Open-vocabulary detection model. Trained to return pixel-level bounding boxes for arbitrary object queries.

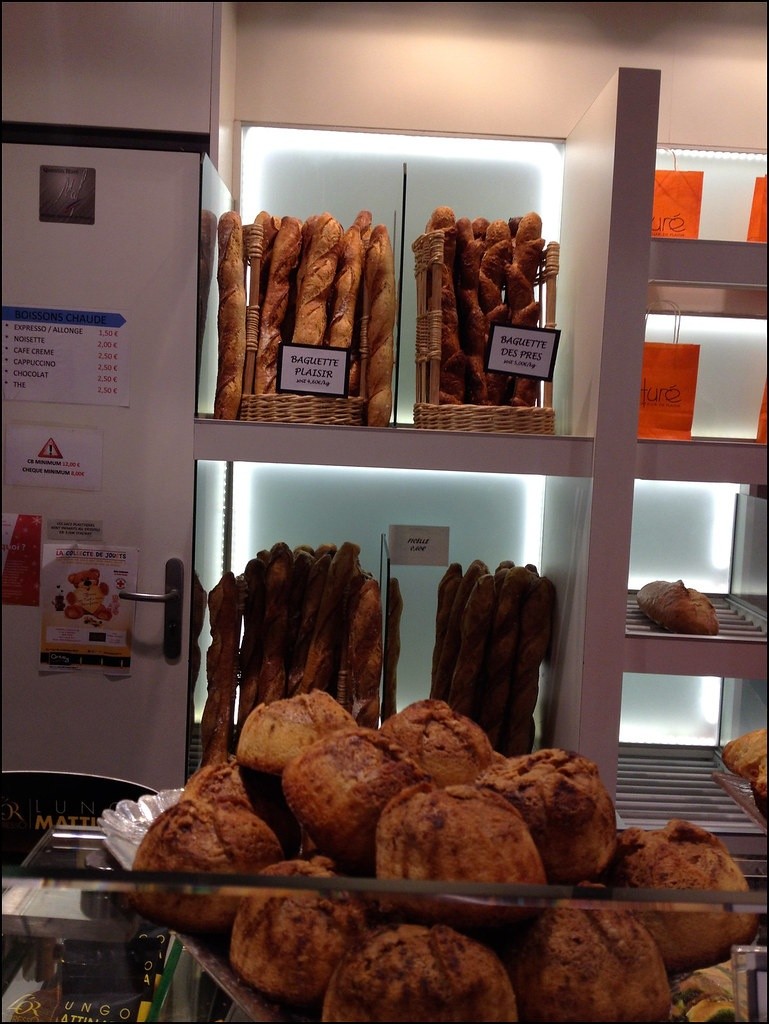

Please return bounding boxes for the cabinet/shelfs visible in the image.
[195,65,769,860]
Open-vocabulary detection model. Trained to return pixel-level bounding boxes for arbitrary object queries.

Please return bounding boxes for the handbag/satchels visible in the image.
[637,299,702,441]
[651,146,705,239]
[746,175,767,244]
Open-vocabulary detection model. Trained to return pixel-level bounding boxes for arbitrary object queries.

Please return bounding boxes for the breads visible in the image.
[637,579,718,637]
[424,205,545,409]
[127,690,751,1021]
[212,208,395,428]
[196,541,403,769]
[426,557,559,757]
[721,727,766,805]
